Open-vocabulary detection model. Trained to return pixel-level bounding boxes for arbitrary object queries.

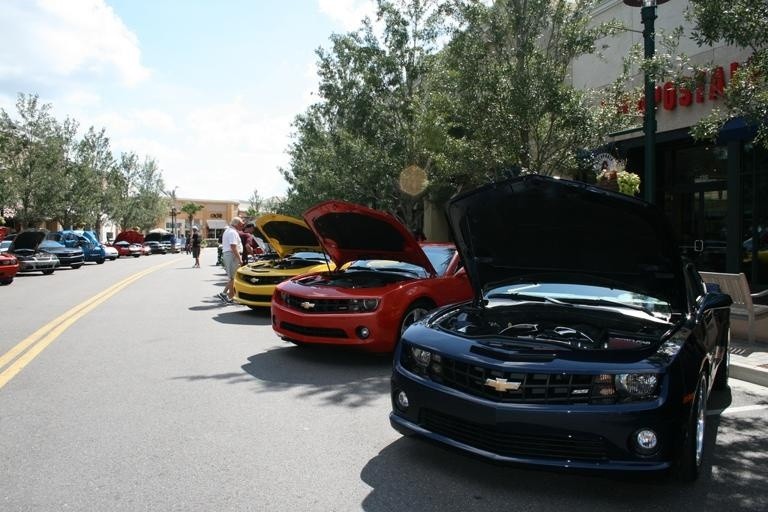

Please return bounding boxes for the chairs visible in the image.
[696,271,767,347]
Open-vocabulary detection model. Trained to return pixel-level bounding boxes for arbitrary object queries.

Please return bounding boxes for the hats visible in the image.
[232,216,245,227]
[192,225,198,231]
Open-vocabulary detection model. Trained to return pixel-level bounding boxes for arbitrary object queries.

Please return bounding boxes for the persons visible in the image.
[238,221,258,266]
[180,233,193,255]
[216,216,245,306]
[189,227,201,269]
[170,234,176,253]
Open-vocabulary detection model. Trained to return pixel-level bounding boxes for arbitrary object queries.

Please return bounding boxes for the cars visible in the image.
[232,213,337,312]
[0,228,184,285]
[269,199,476,362]
[389,173,733,484]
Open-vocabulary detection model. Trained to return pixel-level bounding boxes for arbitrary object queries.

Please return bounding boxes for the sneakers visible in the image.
[218,293,236,304]
